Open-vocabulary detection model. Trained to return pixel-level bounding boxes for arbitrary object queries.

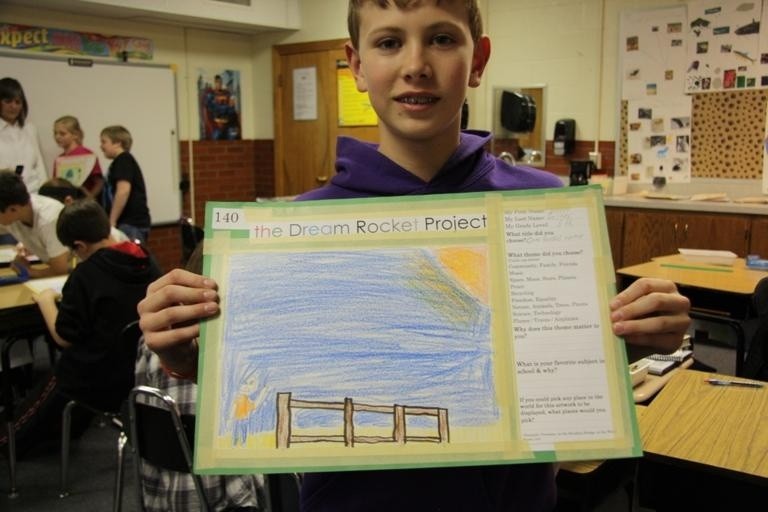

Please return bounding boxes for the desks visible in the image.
[557,360,767,511]
[615,248,768,377]
[0,274,67,342]
[0,236,75,284]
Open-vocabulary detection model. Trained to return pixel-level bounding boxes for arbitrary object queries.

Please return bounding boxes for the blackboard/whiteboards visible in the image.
[0,47,183,229]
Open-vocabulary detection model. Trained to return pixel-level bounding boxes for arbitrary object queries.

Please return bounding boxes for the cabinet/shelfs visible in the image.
[749,216,768,262]
[604,205,624,293]
[622,206,751,269]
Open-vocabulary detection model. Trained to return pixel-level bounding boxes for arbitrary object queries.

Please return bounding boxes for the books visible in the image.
[646,336,691,376]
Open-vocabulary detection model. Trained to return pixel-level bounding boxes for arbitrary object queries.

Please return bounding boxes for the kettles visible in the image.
[569,159,592,187]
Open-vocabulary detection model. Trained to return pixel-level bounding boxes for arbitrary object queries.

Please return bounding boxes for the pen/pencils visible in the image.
[72,256,77,269]
[703,378,763,388]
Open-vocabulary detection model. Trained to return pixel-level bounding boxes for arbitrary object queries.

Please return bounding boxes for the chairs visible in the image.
[115,386,213,511]
[60,319,139,501]
[1,309,52,501]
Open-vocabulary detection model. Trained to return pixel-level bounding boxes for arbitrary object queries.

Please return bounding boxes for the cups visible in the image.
[701,77,711,89]
[723,69,768,89]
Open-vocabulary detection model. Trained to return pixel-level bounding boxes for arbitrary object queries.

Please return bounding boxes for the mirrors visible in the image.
[485,79,549,169]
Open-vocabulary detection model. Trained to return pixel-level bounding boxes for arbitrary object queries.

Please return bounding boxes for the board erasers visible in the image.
[67,58,95,67]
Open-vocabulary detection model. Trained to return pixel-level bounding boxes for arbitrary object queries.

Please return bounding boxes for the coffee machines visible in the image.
[553,118,575,156]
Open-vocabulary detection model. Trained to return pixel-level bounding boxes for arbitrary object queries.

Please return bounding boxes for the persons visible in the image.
[0,77,48,194]
[1,168,132,280]
[52,116,105,200]
[101,125,151,240]
[201,75,239,142]
[38,176,93,205]
[0,202,162,457]
[136,236,272,512]
[136,0,693,512]
